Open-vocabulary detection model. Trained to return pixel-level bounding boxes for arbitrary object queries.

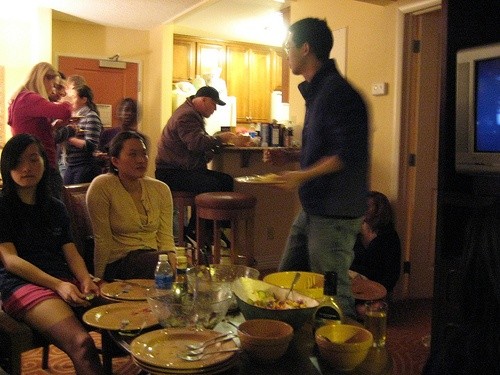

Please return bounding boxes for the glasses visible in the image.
[285,47,295,54]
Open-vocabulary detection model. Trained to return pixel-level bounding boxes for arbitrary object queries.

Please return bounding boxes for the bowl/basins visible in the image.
[186,264,260,297]
[353,280,387,305]
[314,324,373,371]
[237,319,293,364]
[230,136,250,147]
[231,277,319,330]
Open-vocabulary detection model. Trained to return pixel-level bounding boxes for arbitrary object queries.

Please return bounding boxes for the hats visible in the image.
[196,86,226,106]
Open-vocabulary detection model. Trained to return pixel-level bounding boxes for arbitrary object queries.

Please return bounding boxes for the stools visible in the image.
[171,190,198,247]
[195,192,258,267]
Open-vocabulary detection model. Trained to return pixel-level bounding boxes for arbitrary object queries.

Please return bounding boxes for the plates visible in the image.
[129,328,240,375]
[262,271,326,301]
[83,302,159,330]
[233,176,287,184]
[100,279,156,302]
[71,117,85,119]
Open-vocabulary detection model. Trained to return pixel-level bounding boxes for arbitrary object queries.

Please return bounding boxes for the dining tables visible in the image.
[102,310,393,375]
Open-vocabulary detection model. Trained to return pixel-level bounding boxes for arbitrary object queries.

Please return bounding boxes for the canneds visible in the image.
[259,123,283,147]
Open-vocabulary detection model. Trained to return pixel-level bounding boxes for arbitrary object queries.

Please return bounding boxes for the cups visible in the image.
[364,301,387,347]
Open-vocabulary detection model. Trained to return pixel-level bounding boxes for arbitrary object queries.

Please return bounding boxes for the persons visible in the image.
[85,127,177,281]
[348,191,402,311]
[94,98,146,174]
[263,18,370,321]
[155,86,233,248]
[0,133,110,375]
[9,62,102,186]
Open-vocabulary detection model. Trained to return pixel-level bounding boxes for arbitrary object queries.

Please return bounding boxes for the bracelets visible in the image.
[53,280,63,290]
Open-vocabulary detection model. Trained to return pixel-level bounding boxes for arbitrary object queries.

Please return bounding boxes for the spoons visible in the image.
[187,330,232,349]
[187,335,235,355]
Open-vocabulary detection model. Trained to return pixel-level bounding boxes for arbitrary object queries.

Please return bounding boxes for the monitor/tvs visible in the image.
[452,42,500,175]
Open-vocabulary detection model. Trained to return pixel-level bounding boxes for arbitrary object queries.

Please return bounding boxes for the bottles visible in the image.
[261,122,293,147]
[154,254,173,290]
[175,247,188,284]
[312,272,344,341]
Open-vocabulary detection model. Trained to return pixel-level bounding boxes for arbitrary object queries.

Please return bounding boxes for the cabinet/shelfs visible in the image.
[271,46,283,92]
[431,167,500,375]
[173,34,227,84]
[226,40,271,124]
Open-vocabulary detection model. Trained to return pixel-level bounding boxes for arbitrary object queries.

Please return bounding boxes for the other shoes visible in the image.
[186,231,197,248]
[220,232,230,248]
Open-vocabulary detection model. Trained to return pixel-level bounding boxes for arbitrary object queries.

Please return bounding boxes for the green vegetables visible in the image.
[246,290,278,309]
[164,294,222,330]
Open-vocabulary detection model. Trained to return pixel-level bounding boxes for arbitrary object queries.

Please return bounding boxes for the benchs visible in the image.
[0,182,97,375]
